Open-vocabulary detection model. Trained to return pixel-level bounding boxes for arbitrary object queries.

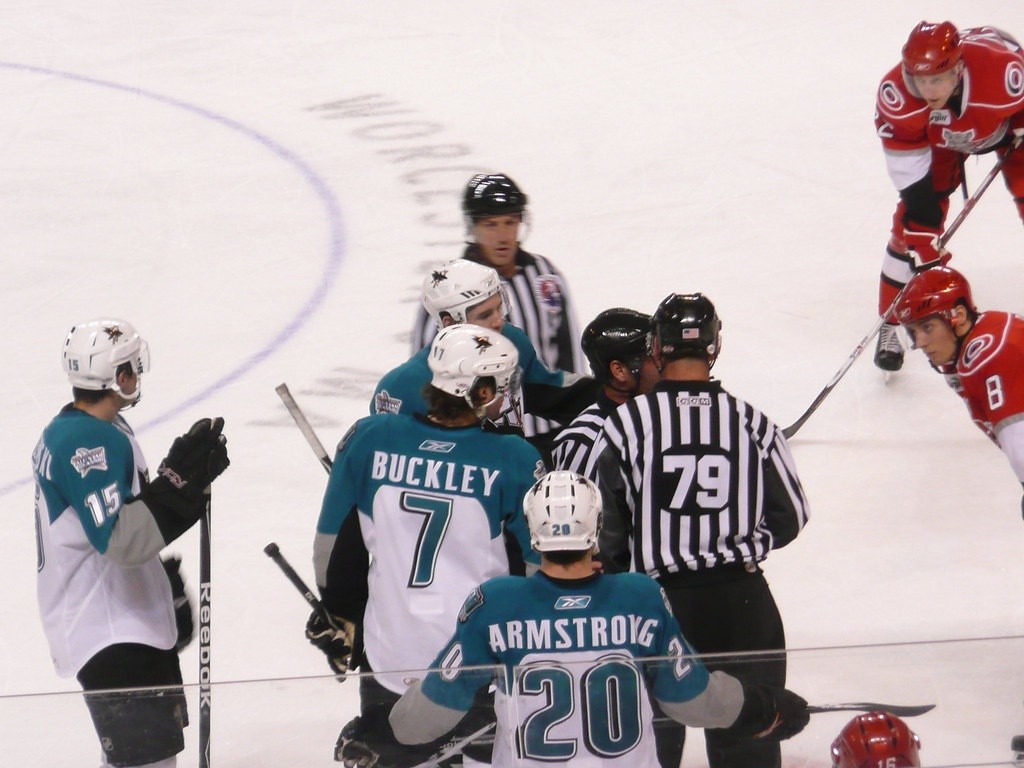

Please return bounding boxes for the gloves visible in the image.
[901,226,951,272]
[157,416,230,500]
[305,601,353,682]
[725,684,809,743]
[163,558,194,655]
[334,705,411,768]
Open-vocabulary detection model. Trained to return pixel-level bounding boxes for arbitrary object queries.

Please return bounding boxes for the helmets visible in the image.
[421,258,500,328]
[63,321,143,402]
[463,173,526,218]
[830,711,919,768]
[427,323,519,397]
[892,265,974,330]
[580,308,655,374]
[523,472,603,555]
[649,291,723,362]
[902,21,962,77]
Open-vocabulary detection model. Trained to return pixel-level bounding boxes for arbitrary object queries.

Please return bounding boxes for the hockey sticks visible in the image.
[448,704,937,749]
[276,383,333,476]
[781,137,1023,441]
[198,484,212,768]
[265,540,338,629]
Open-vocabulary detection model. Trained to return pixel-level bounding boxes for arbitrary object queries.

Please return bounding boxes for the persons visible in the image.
[334,470,811,768]
[33,317,230,768]
[549,309,657,473]
[367,259,583,420]
[305,324,547,768]
[411,174,590,451]
[894,266,1024,520]
[831,710,921,768]
[873,21,1024,386]
[585,293,810,768]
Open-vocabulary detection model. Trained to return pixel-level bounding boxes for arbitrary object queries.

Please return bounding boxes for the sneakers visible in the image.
[874,324,903,382]
